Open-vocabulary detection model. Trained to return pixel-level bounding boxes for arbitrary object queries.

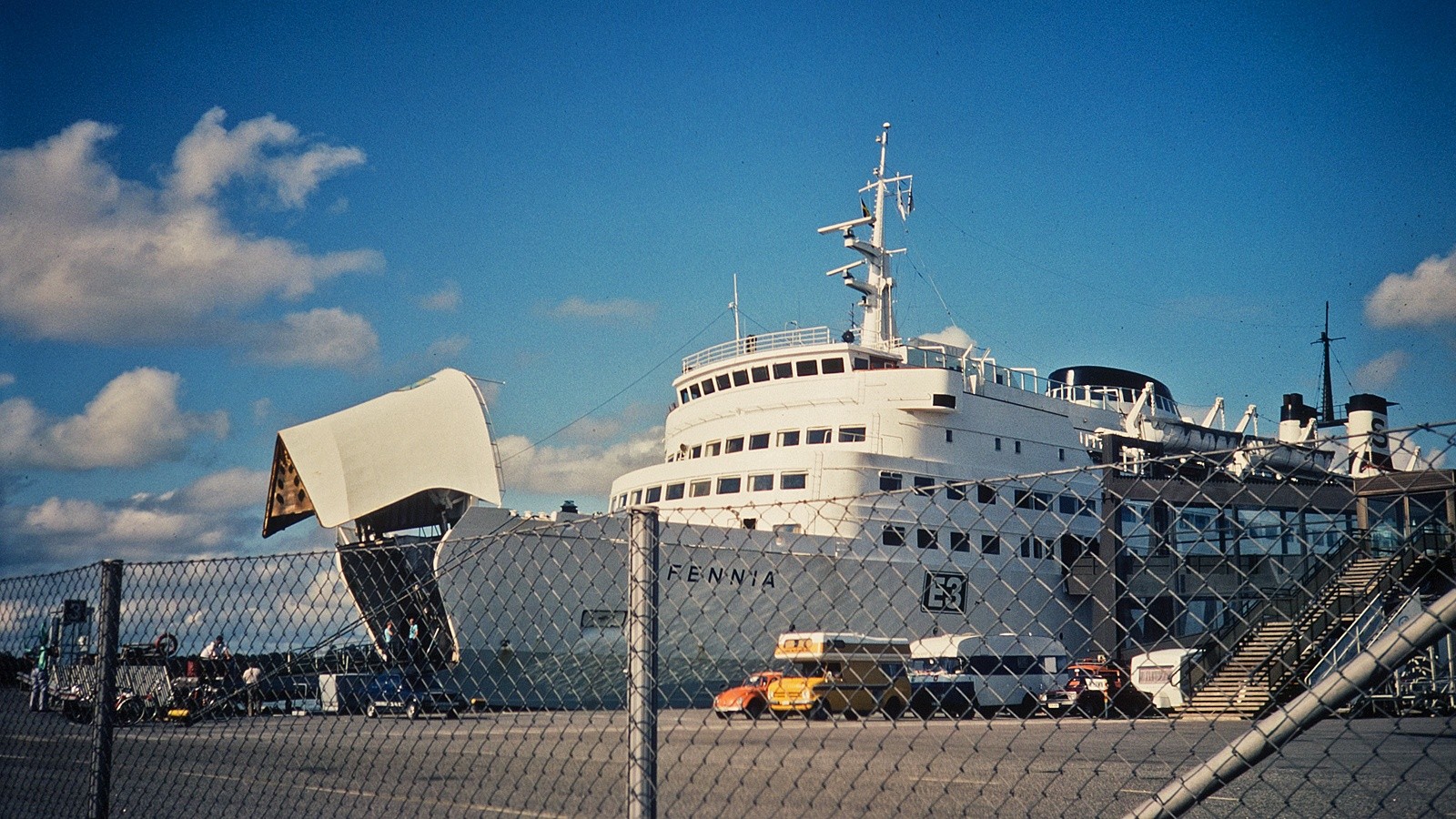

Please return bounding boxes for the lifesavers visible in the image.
[155,633,178,656]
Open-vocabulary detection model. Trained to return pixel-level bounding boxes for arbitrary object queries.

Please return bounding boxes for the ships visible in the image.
[259,122,1456,712]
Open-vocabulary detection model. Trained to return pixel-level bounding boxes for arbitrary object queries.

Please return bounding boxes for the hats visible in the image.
[40,634,48,643]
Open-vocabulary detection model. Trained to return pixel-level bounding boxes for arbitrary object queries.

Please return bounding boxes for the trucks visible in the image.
[764,632,913,721]
[1038,654,1146,720]
[908,632,1071,721]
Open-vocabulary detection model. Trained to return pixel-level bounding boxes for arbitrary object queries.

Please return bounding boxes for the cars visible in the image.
[357,662,470,721]
[713,671,783,719]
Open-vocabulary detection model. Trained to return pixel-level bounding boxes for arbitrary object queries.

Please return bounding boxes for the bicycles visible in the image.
[51,668,238,727]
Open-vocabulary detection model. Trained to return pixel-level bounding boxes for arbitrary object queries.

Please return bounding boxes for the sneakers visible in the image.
[40,710,52,714]
[30,709,40,714]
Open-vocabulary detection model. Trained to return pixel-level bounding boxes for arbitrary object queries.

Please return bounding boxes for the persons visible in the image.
[24,634,59,712]
[384,620,393,661]
[242,662,264,717]
[408,617,418,660]
[200,636,230,660]
